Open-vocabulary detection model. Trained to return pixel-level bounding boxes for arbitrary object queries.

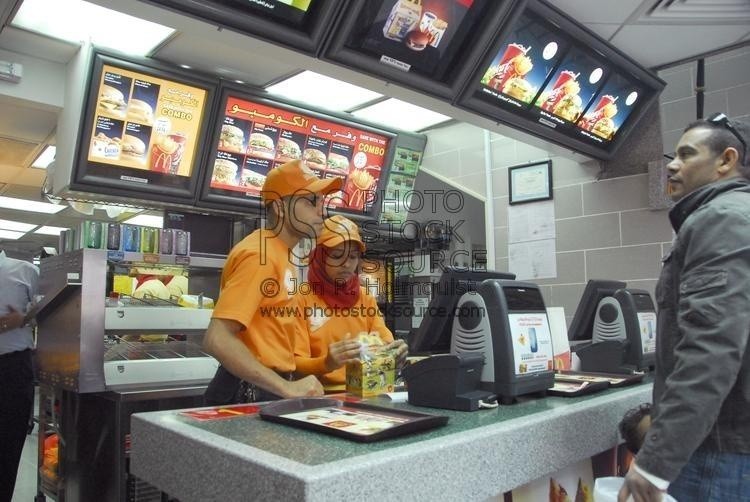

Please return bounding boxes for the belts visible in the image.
[278,373,295,382]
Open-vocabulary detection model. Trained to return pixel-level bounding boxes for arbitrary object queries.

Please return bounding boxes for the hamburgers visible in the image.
[404,31,429,51]
[593,119,615,139]
[552,90,582,120]
[504,78,532,102]
[212,124,351,206]
[87,84,152,167]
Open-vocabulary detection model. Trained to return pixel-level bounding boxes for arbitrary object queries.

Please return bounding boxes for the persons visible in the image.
[1,245,41,501]
[615,111,750,502]
[201,160,325,407]
[294,215,410,396]
[618,402,658,454]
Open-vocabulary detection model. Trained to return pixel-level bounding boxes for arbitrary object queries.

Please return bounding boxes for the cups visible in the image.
[528,327,539,353]
[583,93,615,132]
[540,69,577,115]
[648,320,653,339]
[488,43,527,95]
[420,11,438,32]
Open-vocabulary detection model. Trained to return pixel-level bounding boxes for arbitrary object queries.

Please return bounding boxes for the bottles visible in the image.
[58,219,191,256]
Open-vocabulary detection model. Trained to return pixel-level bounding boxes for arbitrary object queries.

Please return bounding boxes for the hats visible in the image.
[261,161,344,206]
[314,215,366,255]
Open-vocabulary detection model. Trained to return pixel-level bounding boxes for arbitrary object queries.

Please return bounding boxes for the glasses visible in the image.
[708,110,747,166]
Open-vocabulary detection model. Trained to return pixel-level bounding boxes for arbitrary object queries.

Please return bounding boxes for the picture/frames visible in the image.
[507,160,553,205]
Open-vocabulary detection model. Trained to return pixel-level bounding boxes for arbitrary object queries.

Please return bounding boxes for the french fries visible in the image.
[603,101,618,119]
[433,18,447,30]
[348,168,373,188]
[152,129,179,154]
[564,80,581,95]
[514,56,534,75]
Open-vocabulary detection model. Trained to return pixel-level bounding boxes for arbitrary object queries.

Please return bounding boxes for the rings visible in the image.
[3,324,8,330]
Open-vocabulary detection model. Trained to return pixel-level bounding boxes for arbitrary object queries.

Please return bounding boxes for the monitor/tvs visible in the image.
[567,280,627,341]
[406,266,516,357]
